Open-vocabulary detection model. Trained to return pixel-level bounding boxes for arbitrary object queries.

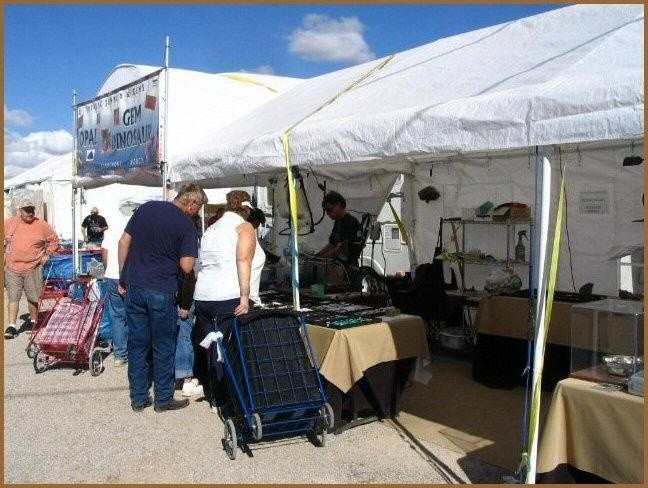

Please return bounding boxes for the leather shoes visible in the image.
[153,398,190,413]
[131,396,152,412]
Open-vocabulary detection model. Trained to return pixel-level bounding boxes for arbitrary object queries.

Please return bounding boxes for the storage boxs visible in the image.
[437,326,473,349]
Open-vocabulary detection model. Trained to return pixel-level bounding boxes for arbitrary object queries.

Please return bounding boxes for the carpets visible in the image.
[353,351,553,475]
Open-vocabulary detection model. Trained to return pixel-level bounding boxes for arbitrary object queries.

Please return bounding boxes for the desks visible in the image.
[472,289,645,385]
[252,289,431,434]
[534,354,644,483]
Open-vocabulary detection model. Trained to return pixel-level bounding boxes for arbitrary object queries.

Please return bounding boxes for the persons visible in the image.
[114,181,208,413]
[243,206,267,232]
[188,190,268,415]
[102,203,134,366]
[174,211,204,399]
[315,189,367,270]
[80,206,109,248]
[3,196,60,341]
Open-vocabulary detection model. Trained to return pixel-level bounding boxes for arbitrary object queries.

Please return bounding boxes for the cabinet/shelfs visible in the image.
[436,217,531,316]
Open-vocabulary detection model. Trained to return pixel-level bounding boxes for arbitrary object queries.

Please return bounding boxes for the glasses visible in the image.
[326,204,338,213]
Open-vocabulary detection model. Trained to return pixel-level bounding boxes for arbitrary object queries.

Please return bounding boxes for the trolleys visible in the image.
[30,261,116,377]
[213,306,336,459]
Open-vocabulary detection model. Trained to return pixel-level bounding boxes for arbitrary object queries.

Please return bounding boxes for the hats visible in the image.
[242,201,253,209]
[19,198,35,209]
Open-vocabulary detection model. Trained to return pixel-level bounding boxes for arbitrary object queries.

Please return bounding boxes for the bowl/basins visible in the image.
[600,354,643,376]
[439,327,468,349]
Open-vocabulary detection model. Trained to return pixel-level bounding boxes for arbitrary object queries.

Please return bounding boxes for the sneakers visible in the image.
[4,326,19,338]
[25,321,39,334]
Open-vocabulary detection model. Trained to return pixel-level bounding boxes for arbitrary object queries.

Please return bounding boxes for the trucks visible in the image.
[359,174,410,295]
